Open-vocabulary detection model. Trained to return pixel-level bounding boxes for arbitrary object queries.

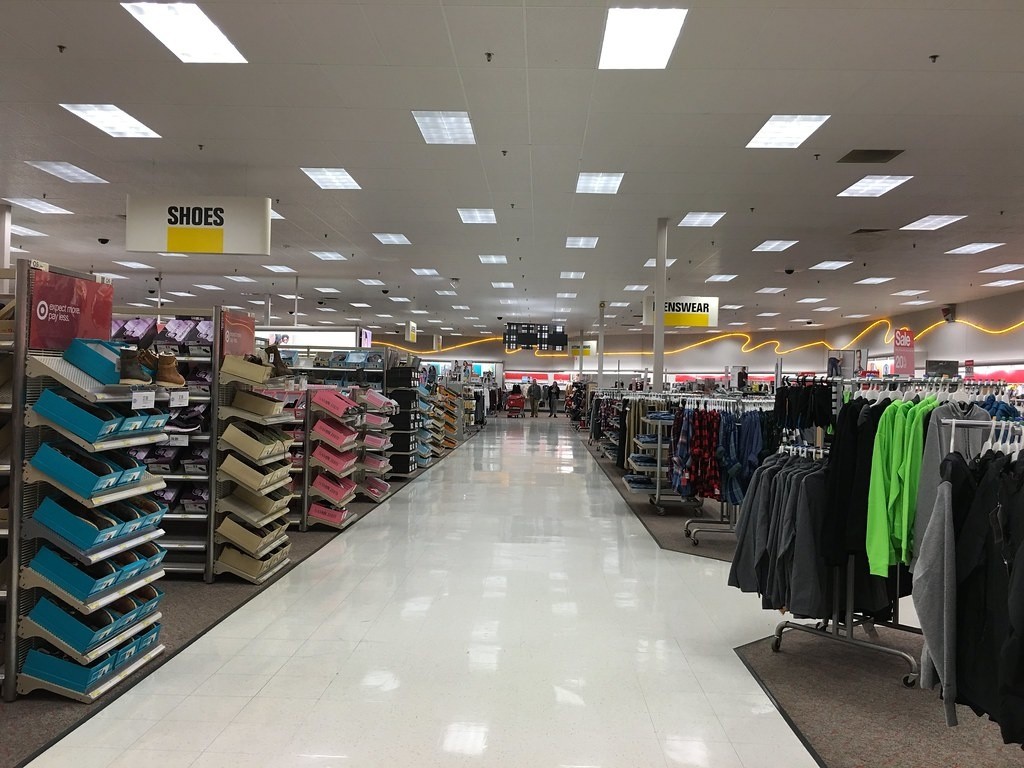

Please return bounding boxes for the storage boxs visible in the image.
[20,316,459,696]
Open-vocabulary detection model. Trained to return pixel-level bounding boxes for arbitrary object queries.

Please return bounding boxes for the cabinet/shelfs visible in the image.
[434,393,446,457]
[622,410,705,516]
[417,389,434,469]
[0,259,188,705]
[339,384,400,504]
[254,364,359,532]
[439,385,459,449]
[112,304,291,585]
[277,345,427,480]
[597,402,624,459]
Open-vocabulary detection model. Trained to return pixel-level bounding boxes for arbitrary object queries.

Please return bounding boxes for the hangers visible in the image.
[948,418,957,453]
[778,442,825,461]
[783,374,1024,416]
[980,417,1024,462]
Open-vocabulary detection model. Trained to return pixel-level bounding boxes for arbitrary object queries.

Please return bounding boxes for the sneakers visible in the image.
[159,406,200,432]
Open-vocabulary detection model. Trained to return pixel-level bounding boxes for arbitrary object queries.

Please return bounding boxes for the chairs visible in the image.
[590,385,775,417]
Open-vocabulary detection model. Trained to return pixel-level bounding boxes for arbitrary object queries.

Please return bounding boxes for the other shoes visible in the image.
[535,414,538,417]
[554,415,558,418]
[27,388,170,668]
[549,414,553,417]
[530,413,534,417]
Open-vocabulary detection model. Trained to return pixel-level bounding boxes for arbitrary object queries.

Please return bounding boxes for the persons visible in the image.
[829,351,843,376]
[854,350,864,372]
[454,360,460,371]
[527,379,541,418]
[428,366,436,383]
[547,381,560,418]
[738,366,748,390]
[463,361,470,378]
[420,368,427,383]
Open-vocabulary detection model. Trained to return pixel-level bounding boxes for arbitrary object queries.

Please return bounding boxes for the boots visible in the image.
[137,348,159,372]
[155,350,185,388]
[119,346,153,386]
